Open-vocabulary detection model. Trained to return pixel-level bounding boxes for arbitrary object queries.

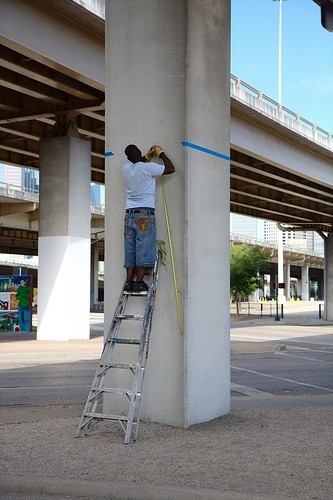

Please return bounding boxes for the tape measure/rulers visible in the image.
[151,146,183,334]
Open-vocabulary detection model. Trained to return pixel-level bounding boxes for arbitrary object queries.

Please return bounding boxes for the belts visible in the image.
[125,209,154,215]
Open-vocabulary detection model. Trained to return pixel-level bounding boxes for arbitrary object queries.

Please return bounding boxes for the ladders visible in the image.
[76,240,165,445]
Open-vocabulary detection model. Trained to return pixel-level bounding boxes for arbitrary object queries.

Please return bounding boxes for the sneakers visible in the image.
[122,280,135,294]
[132,280,149,296]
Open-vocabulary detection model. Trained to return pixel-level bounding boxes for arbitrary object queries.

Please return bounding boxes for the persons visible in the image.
[122,144,175,293]
[15,279,30,332]
